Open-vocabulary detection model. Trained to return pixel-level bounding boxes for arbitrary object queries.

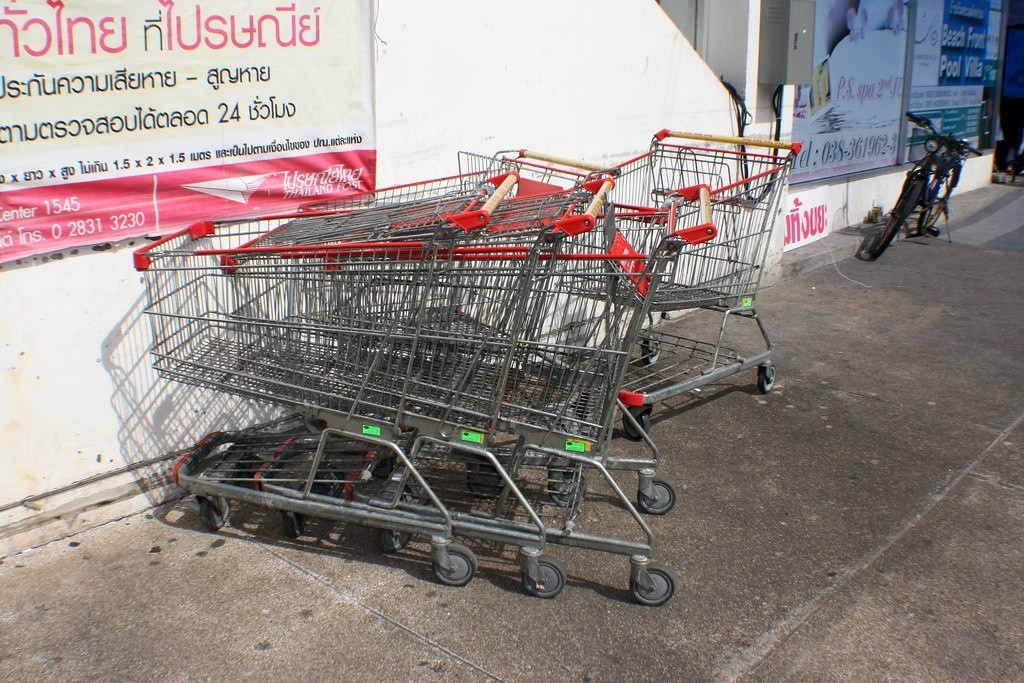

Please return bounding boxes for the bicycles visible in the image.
[865,111,986,255]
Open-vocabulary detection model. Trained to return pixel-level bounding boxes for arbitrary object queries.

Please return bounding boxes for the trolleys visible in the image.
[133,130,804,604]
